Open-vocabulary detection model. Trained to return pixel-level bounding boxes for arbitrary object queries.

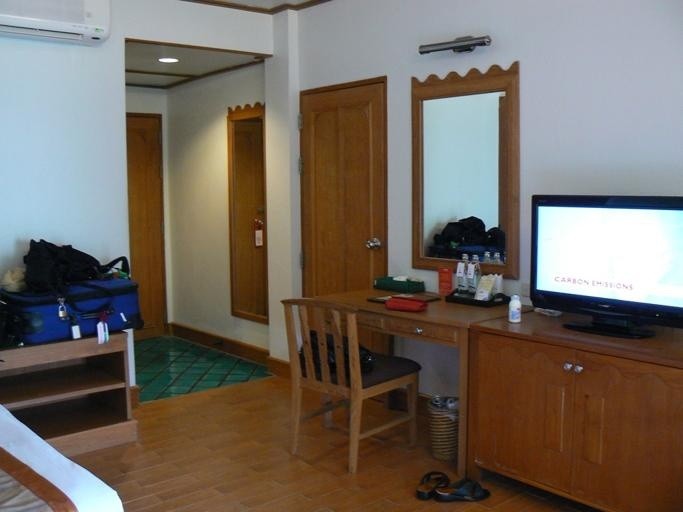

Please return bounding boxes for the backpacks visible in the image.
[20,239,130,316]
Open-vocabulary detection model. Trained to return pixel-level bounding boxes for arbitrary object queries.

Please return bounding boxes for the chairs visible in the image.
[279,298,422,475]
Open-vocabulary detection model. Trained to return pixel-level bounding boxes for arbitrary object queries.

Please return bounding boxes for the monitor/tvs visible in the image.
[530,194,683,340]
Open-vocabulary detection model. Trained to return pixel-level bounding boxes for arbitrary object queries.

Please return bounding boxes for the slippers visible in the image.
[431,477,491,503]
[415,471,450,501]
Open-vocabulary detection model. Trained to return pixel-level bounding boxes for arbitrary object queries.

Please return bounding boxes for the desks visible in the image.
[314,288,533,479]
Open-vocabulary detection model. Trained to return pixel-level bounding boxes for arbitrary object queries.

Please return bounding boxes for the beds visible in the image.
[0,403,125,512]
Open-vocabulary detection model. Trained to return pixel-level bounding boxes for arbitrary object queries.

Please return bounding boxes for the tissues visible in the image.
[375,275,425,293]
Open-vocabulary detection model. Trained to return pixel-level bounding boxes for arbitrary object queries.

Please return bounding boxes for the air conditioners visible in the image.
[1,0,111,47]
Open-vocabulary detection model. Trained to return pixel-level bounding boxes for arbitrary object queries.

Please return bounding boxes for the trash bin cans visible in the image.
[426,396,460,461]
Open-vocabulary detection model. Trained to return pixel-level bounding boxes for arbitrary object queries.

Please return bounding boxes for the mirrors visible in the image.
[409,60,521,280]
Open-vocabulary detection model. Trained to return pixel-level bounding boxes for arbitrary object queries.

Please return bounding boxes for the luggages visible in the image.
[428,245,505,263]
[0,267,143,347]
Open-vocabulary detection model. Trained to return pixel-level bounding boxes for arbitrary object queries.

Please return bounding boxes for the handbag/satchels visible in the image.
[434,216,505,246]
[301,329,376,372]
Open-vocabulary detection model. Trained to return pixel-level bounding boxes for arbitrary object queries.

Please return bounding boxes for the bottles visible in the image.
[455,253,481,293]
[507,294,522,324]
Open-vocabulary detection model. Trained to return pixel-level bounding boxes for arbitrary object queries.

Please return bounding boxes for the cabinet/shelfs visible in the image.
[0,332,138,458]
[467,308,683,512]
